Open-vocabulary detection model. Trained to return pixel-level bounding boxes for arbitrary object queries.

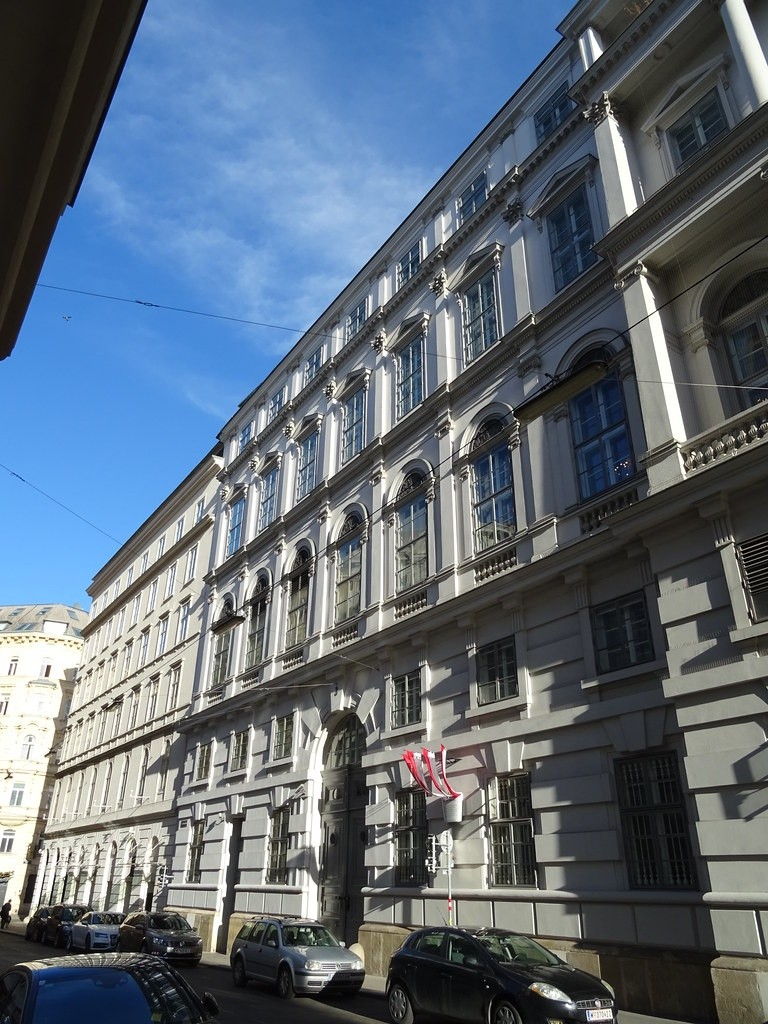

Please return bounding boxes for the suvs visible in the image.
[45,904,103,950]
[119,910,204,971]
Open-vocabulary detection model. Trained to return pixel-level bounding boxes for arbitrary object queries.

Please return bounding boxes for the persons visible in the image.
[0,899,12,929]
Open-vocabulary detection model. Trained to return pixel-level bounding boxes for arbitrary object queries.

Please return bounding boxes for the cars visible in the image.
[229,915,367,1002]
[66,911,126,955]
[0,950,222,1024]
[26,906,73,945]
[386,923,620,1024]
